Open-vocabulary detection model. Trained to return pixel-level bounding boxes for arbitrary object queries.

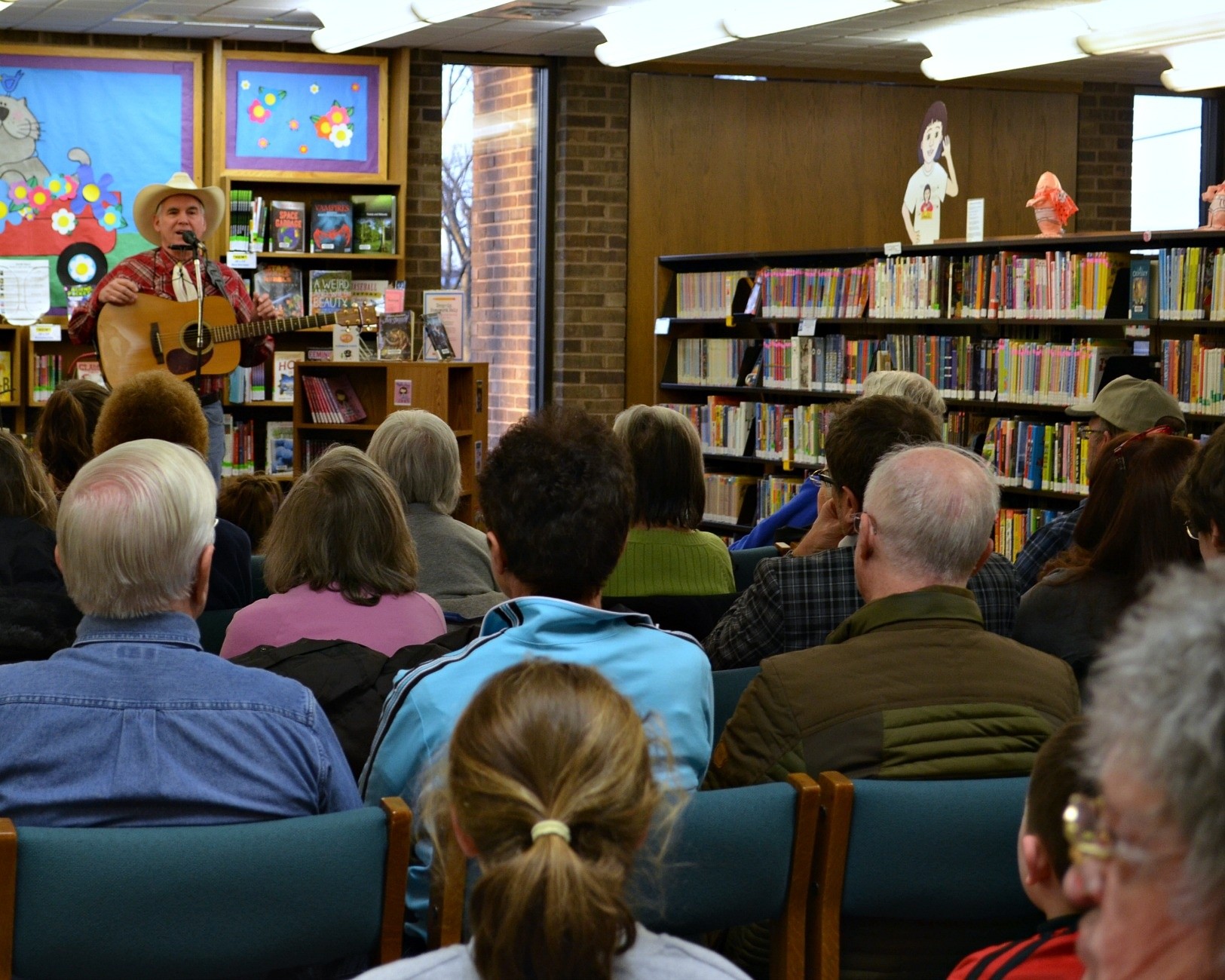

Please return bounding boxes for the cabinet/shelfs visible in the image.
[0,317,97,448]
[294,361,488,540]
[649,229,1225,567]
[214,178,407,482]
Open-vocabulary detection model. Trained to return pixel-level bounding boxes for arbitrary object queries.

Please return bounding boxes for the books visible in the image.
[677,247,1225,563]
[230,191,397,254]
[219,267,466,475]
[1,351,109,402]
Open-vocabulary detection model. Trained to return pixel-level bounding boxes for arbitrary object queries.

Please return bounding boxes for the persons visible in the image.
[66,173,278,487]
[0,371,1225,980]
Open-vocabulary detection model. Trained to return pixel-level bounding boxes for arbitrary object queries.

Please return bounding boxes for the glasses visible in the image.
[1077,424,1103,440]
[1057,795,1201,903]
[807,468,842,488]
[1114,424,1174,478]
[1183,518,1203,542]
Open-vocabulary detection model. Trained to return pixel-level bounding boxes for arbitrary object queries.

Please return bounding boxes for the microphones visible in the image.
[182,230,207,253]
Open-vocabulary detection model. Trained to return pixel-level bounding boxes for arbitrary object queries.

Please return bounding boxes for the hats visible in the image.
[1066,374,1187,432]
[132,170,226,248]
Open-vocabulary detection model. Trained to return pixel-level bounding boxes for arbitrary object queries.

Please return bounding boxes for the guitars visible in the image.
[90,291,383,394]
